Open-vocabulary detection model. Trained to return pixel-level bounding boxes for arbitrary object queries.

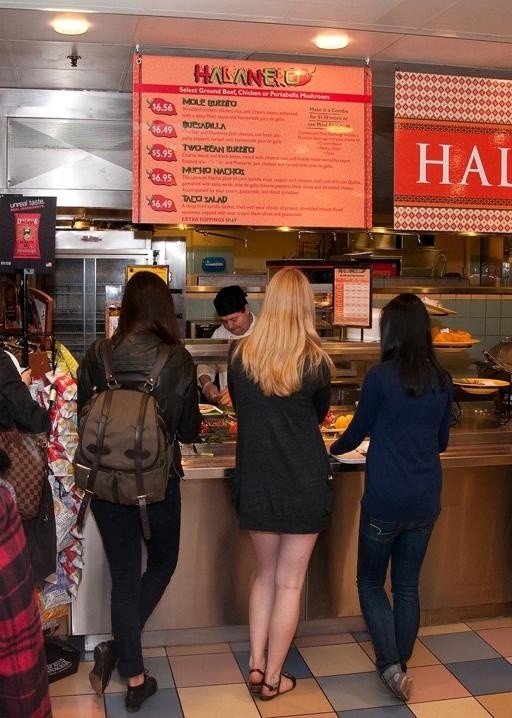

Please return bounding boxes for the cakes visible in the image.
[431,324,471,342]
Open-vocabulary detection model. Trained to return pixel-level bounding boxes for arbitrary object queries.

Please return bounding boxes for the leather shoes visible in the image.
[120,670,159,715]
[85,637,117,697]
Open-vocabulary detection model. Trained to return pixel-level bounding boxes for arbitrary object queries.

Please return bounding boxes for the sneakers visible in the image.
[379,662,415,703]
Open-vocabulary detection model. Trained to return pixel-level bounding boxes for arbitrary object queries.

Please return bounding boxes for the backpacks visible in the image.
[70,336,180,507]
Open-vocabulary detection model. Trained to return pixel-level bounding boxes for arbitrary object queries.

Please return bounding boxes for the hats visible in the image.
[211,284,248,318]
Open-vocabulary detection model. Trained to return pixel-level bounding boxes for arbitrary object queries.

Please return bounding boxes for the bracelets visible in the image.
[201,380,213,394]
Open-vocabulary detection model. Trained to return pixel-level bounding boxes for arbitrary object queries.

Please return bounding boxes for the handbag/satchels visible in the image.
[0,427,50,522]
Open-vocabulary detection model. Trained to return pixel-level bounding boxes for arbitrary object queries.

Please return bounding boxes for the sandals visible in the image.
[258,671,296,702]
[246,665,264,695]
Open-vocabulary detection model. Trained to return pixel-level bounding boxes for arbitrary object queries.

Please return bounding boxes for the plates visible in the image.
[451,377,511,395]
[431,338,481,348]
[323,440,370,465]
[197,403,215,413]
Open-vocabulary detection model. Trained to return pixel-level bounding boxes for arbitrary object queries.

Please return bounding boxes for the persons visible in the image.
[330,293,453,699]
[195,284,262,408]
[70,270,208,714]
[225,266,336,699]
[0,348,58,582]
